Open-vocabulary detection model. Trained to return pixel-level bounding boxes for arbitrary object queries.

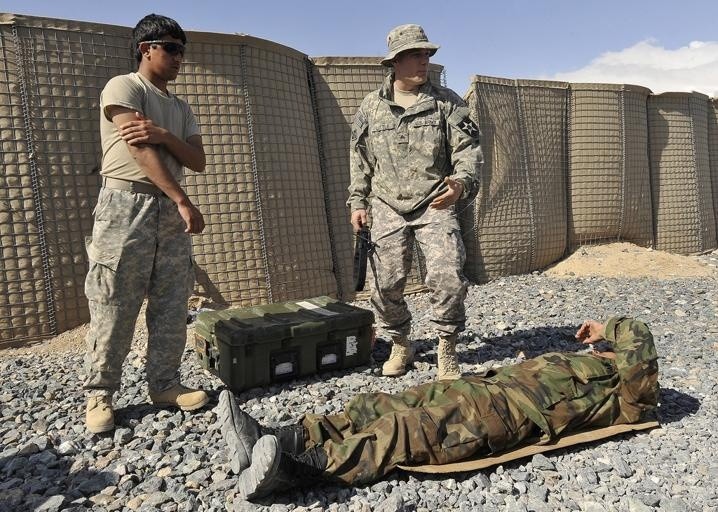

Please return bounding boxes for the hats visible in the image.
[380,24,440,67]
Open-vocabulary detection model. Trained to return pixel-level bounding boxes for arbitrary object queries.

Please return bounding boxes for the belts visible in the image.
[101,175,166,196]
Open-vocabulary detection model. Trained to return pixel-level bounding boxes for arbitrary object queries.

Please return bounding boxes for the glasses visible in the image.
[137,40,186,57]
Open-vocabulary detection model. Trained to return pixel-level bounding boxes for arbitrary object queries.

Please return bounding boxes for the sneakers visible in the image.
[151,383,210,411]
[85,395,115,433]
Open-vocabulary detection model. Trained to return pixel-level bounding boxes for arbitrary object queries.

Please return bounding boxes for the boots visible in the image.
[237,434,328,500]
[382,334,415,376]
[217,389,309,474]
[437,336,462,380]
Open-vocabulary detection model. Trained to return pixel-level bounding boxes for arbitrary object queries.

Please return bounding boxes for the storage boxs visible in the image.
[194,294,376,395]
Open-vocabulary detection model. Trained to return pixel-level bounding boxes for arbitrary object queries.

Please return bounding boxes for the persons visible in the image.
[217,315,661,501]
[345,23,486,382]
[81,14,210,435]
